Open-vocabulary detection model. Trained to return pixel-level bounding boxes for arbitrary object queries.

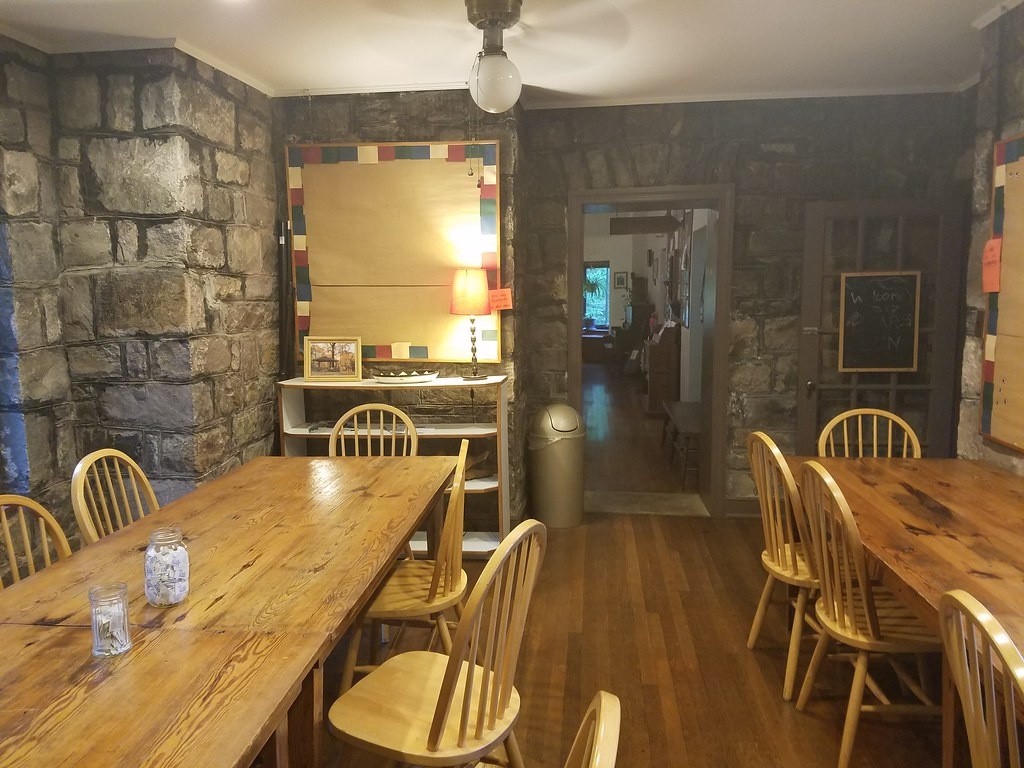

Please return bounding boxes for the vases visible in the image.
[585,319,595,328]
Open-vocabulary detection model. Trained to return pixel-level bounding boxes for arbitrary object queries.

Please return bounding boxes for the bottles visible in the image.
[87,582,134,658]
[143,526,192,609]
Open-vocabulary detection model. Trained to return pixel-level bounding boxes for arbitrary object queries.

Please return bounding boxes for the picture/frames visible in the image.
[614,271,628,289]
[304,336,362,382]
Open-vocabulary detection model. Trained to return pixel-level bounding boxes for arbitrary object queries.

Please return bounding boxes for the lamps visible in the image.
[450,269,491,380]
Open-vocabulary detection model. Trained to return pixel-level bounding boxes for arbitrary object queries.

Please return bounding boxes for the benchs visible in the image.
[661,399,700,492]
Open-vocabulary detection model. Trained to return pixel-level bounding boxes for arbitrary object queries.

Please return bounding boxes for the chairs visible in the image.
[338,439,483,697]
[564,690,621,768]
[329,403,418,560]
[328,519,548,768]
[747,408,1024,768]
[71,448,159,550]
[0,494,72,590]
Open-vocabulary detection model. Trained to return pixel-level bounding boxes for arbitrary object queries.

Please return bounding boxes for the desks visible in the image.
[0,456,459,767]
[768,454,1024,768]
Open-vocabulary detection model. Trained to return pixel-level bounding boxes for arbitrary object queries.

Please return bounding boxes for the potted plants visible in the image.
[583,279,607,299]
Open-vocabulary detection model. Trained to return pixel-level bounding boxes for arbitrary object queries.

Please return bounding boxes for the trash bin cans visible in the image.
[528,403,589,530]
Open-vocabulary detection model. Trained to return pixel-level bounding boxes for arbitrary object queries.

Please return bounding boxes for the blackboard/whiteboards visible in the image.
[838,268,923,374]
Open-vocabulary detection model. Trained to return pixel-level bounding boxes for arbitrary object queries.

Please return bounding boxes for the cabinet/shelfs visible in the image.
[277,375,510,560]
[643,322,681,414]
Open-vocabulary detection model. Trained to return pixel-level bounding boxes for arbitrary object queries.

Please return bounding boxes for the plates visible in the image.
[374,370,439,383]
